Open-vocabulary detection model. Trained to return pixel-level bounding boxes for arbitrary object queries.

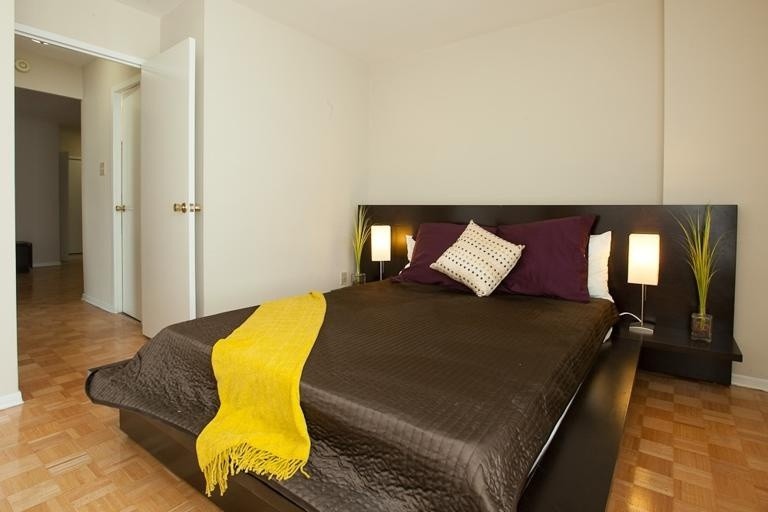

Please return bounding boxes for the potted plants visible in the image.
[668,201,730,343]
[347,200,372,286]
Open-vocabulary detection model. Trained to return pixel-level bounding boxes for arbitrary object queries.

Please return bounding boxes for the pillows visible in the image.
[390,213,617,307]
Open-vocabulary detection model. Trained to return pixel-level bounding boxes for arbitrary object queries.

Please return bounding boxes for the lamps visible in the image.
[369,224,392,281]
[626,232,663,335]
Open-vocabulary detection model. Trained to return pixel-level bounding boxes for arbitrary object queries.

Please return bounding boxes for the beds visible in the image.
[151,274,622,510]
